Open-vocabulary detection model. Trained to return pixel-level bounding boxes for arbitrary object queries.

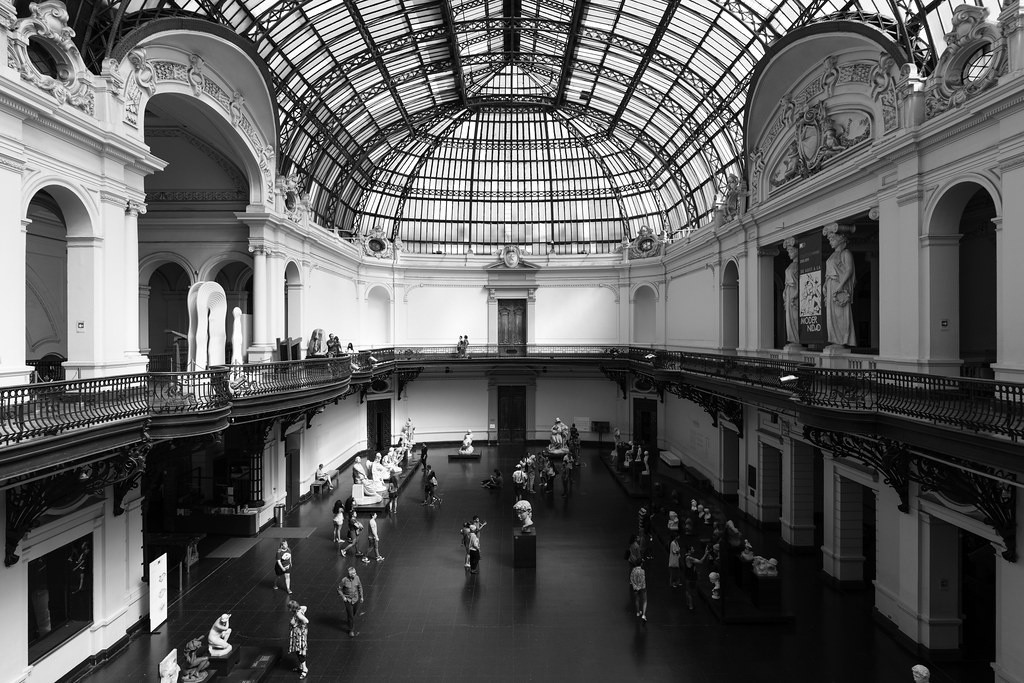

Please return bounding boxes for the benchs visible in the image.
[310,469,339,495]
[683,466,709,492]
[659,450,681,468]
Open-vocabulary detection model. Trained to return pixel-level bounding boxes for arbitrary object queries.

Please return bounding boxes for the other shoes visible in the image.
[286,590,292,594]
[637,611,642,616]
[390,509,398,514]
[439,499,442,504]
[362,558,370,563]
[544,483,547,487]
[420,501,427,505]
[334,539,345,544]
[273,585,278,590]
[341,549,346,557]
[355,551,363,555]
[464,563,471,567]
[428,503,434,507]
[642,615,647,621]
[375,555,385,562]
[538,483,543,485]
[527,491,537,494]
[669,582,682,588]
[470,570,479,574]
[349,630,360,637]
[434,498,438,502]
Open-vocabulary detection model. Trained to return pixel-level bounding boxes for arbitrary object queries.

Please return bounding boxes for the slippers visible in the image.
[300,669,308,679]
[292,667,303,672]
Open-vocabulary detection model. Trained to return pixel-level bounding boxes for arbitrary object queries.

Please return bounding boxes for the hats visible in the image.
[470,524,477,531]
[515,464,522,468]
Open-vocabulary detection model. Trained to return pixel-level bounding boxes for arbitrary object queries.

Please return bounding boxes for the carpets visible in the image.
[256,526,318,539]
[204,537,263,558]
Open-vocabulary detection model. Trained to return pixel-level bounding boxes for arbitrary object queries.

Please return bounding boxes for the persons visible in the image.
[666,499,779,601]
[317,463,334,491]
[548,417,569,451]
[912,665,930,683]
[272,538,293,594]
[513,499,534,529]
[420,423,710,624]
[822,223,859,349]
[287,600,308,679]
[337,566,364,636]
[183,635,211,678]
[456,336,464,354]
[782,236,800,348]
[209,614,233,655]
[460,335,470,357]
[460,429,474,451]
[331,496,385,563]
[352,417,416,503]
[326,333,354,376]
[387,475,400,514]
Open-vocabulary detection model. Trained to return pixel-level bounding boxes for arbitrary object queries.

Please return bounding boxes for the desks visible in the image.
[203,506,260,537]
